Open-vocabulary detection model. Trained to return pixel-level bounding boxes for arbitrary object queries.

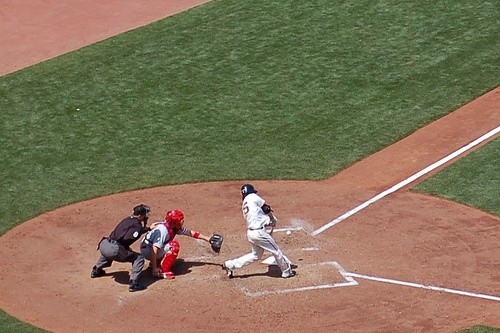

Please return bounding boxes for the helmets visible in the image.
[166,209,184,230]
[133,204,150,228]
[241,184,257,196]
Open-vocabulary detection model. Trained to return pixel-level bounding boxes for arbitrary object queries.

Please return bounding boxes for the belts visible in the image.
[141,246,146,249]
[250,227,263,230]
[107,238,117,243]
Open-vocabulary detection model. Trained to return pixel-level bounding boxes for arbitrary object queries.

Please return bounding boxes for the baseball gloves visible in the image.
[211,234,223,253]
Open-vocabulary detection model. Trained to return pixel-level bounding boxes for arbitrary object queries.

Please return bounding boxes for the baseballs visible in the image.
[285,230,292,235]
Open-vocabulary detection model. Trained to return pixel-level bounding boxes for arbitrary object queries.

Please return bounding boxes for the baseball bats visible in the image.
[269,226,273,237]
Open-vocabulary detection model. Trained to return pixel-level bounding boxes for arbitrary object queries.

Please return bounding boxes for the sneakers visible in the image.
[159,272,174,279]
[222,263,233,278]
[90,270,105,278]
[129,282,145,292]
[288,271,296,277]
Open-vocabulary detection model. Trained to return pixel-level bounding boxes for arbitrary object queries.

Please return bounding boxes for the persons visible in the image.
[222,184,296,278]
[140,210,223,279]
[91,203,160,292]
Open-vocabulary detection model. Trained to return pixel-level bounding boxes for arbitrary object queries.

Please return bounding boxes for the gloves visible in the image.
[269,223,276,227]
[272,217,277,223]
